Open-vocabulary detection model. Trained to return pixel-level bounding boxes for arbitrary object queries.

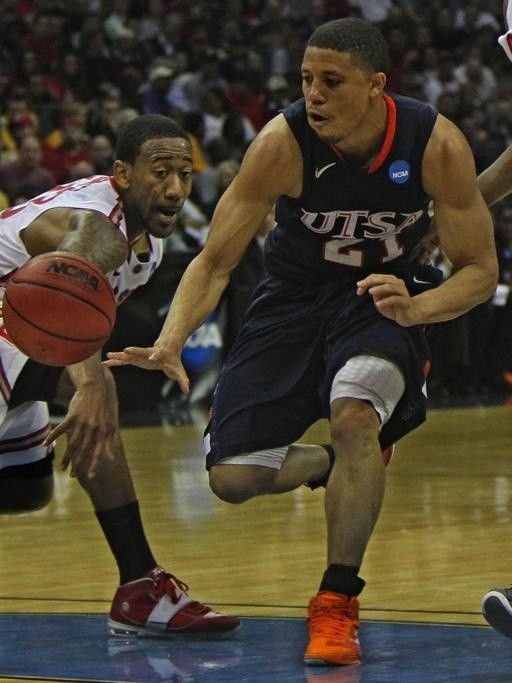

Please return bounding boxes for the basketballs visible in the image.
[2,251,116,366]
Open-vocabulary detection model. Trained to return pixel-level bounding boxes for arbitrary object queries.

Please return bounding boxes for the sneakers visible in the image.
[482,585,510,638]
[378,443,395,468]
[303,590,364,665]
[107,564,241,643]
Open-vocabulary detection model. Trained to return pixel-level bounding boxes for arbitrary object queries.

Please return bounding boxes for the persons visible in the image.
[0,111,244,642]
[410,0,512,651]
[95,15,499,670]
